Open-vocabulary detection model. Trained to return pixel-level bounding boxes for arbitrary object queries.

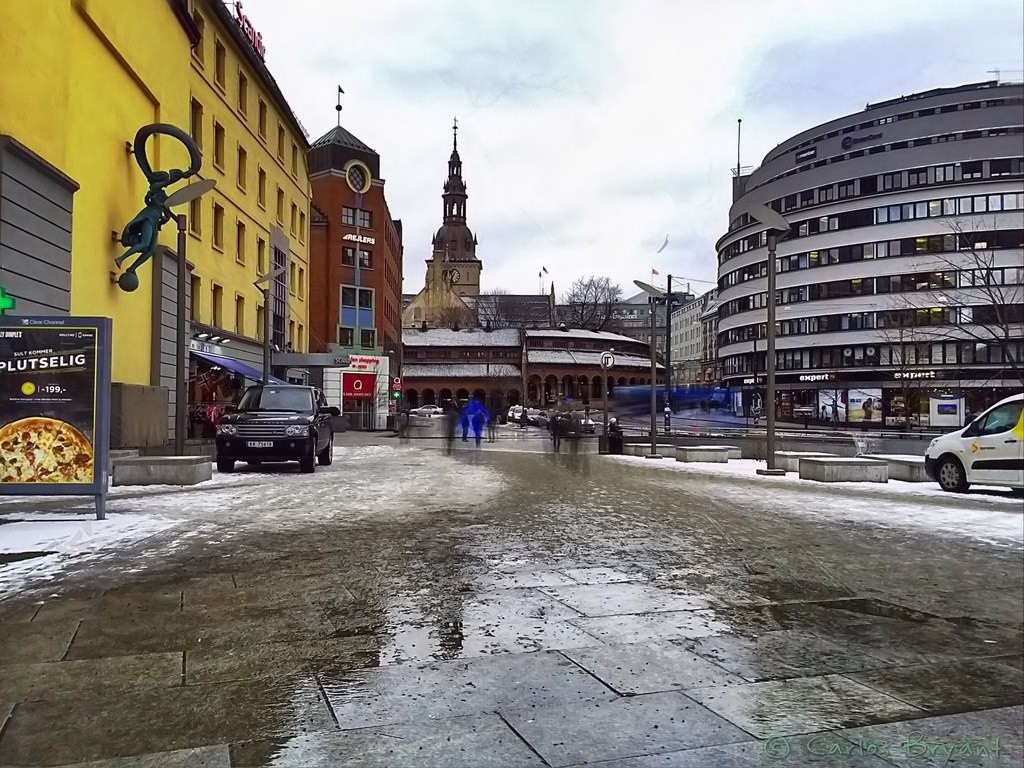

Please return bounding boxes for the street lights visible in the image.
[633,280,664,459]
[252,266,289,384]
[742,200,792,477]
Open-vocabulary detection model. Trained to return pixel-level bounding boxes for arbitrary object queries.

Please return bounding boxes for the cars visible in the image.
[409,404,444,415]
[507,404,596,434]
[925,393,1024,493]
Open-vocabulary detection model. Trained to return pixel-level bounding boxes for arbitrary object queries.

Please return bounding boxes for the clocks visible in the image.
[451,269,461,283]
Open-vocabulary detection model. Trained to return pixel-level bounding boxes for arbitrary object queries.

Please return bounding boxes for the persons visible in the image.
[549,410,563,450]
[610,417,619,437]
[461,406,498,445]
[819,400,844,422]
[862,397,875,420]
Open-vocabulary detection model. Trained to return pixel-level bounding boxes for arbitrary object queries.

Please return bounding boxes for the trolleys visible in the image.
[852,433,884,458]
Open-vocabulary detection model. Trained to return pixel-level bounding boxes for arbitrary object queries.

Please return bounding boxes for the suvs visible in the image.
[215,384,341,473]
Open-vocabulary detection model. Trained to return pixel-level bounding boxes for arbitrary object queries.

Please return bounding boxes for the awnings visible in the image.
[190,351,293,385]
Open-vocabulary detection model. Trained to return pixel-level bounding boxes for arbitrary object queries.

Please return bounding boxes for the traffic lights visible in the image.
[391,391,401,399]
